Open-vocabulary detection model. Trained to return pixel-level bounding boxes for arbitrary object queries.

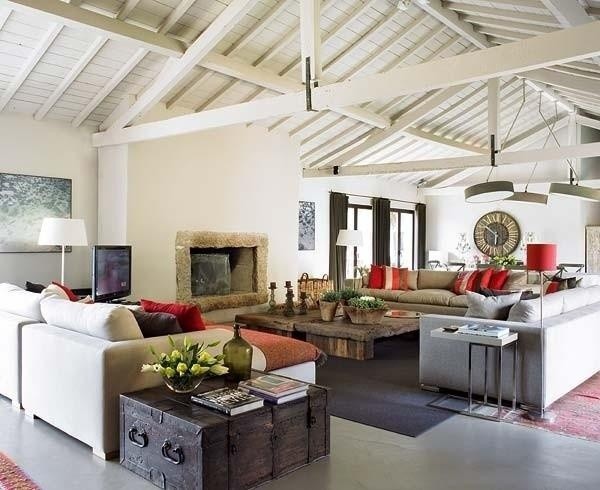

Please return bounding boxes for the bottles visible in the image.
[223,324,253,380]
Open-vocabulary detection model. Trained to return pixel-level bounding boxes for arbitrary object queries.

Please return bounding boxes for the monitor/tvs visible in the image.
[91,243,132,303]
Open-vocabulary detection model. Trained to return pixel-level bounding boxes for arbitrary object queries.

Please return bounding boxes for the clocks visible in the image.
[473,210,522,258]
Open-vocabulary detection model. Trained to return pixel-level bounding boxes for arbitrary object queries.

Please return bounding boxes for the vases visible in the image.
[162,375,206,394]
[342,306,388,326]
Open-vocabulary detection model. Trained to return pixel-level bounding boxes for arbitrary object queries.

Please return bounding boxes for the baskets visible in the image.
[299,272,334,310]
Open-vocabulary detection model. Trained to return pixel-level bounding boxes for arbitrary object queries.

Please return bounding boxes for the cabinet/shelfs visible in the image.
[427,325,518,420]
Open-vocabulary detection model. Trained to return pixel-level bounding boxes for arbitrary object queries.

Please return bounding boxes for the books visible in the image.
[190,386,265,416]
[237,373,310,405]
[430,322,518,346]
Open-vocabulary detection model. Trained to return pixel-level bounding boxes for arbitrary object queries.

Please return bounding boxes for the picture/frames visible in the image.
[0,173,73,254]
[298,201,315,251]
[585,224,600,275]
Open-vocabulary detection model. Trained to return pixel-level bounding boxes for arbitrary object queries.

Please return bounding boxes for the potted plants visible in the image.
[339,287,362,319]
[319,292,340,322]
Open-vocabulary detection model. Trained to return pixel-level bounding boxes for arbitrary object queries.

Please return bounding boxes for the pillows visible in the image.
[39,282,206,338]
[543,274,583,297]
[367,264,418,291]
[450,269,533,319]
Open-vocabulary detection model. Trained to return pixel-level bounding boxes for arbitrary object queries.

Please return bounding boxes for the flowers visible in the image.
[137,333,231,378]
[360,295,375,308]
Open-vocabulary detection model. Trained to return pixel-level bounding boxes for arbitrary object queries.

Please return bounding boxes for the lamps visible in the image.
[464,76,526,204]
[502,99,559,206]
[36,217,89,286]
[335,229,363,247]
[536,90,600,203]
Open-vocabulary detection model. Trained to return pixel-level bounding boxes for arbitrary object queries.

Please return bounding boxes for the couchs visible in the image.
[343,263,539,316]
[419,269,599,422]
[0,282,319,460]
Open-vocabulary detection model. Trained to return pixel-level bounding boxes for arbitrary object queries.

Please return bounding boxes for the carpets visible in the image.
[316,340,478,438]
[0,451,41,490]
[465,372,599,445]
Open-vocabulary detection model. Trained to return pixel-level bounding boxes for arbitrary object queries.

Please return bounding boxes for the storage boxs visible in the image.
[118,365,330,490]
